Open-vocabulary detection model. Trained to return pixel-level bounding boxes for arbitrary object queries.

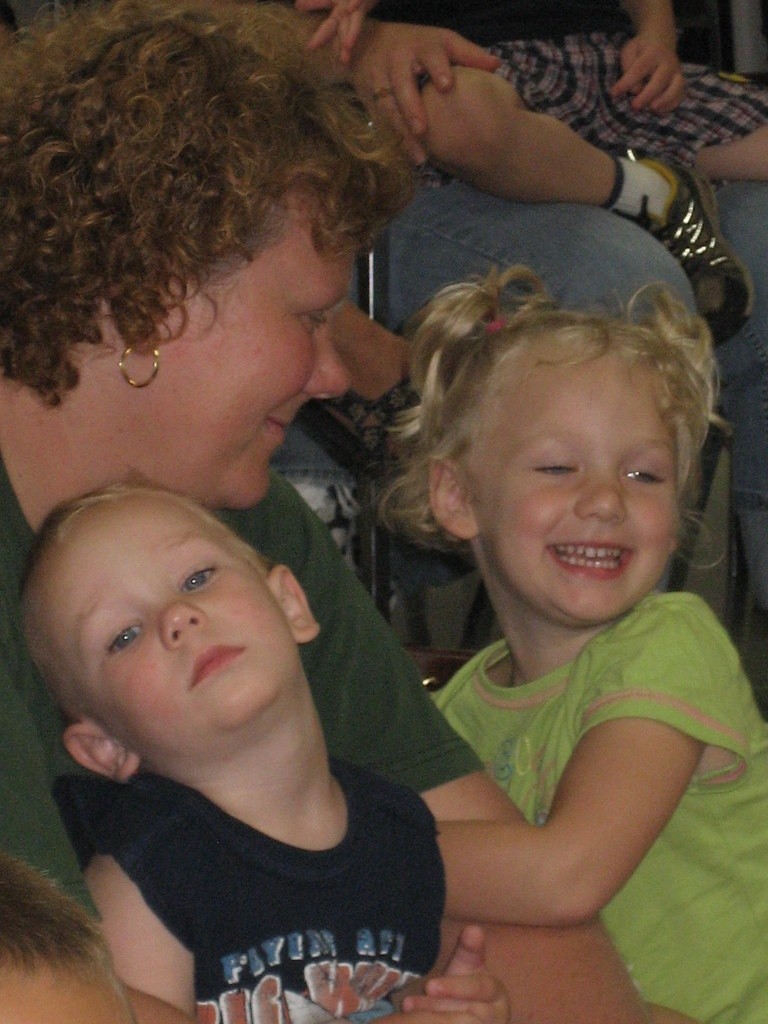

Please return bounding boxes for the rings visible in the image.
[373,88,392,102]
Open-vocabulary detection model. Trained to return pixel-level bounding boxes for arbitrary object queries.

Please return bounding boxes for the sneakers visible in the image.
[627,149,754,347]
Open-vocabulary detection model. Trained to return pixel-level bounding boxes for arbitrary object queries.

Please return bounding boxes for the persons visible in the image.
[0,0,691,1024]
[0,854,133,1024]
[298,0,768,652]
[398,268,768,1023]
[15,482,510,1023]
